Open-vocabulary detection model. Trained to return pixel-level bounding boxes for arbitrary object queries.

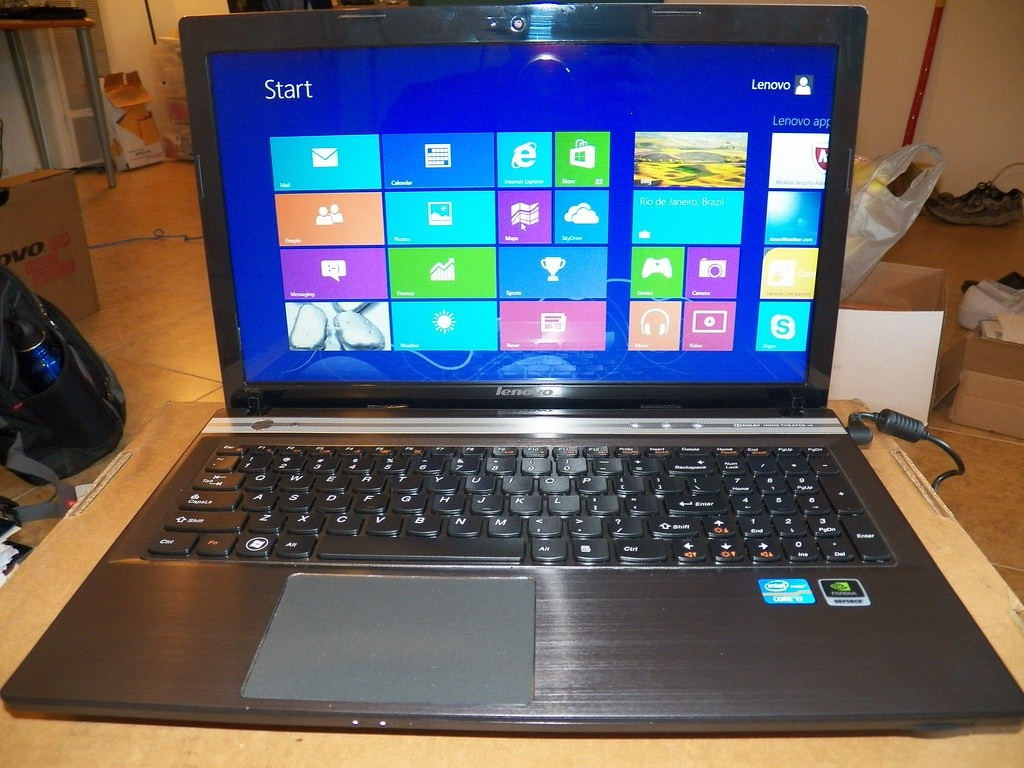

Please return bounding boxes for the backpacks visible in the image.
[0,260,127,532]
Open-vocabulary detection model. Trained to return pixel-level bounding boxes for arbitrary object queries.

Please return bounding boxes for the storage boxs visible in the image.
[827,261,948,428]
[931,331,1023,439]
[97,36,196,172]
[0,167,102,323]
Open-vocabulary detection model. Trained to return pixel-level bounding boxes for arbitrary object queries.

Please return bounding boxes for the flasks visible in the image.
[12,318,63,391]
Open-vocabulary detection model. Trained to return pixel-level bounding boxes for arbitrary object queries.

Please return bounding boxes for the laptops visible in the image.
[0,0,1024,738]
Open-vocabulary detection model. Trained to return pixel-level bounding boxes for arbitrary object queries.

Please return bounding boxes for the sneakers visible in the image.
[926,180,1024,226]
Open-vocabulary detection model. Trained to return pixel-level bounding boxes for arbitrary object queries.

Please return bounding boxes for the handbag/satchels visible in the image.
[838,142,945,302]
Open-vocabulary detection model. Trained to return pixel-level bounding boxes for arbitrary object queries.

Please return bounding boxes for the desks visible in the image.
[1,17,117,189]
[1,400,1023,768]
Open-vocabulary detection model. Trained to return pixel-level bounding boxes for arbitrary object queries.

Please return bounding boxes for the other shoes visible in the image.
[960,271,1024,295]
[956,276,1024,330]
